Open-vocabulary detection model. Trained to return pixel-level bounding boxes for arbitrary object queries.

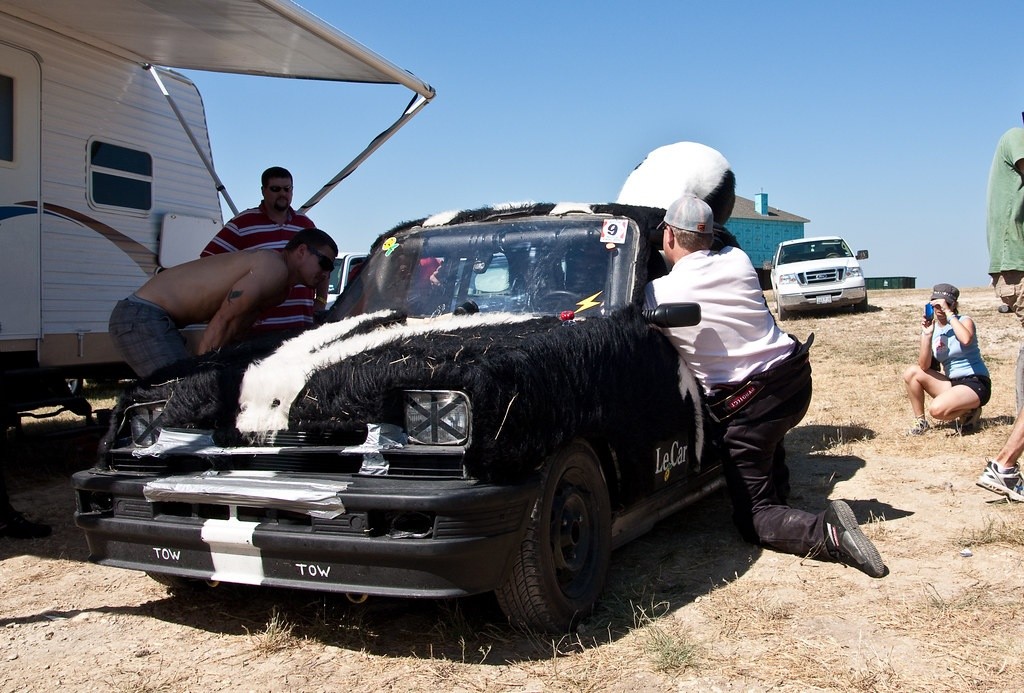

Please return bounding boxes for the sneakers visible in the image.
[957,406,984,433]
[0,503,51,539]
[823,501,884,578]
[908,421,929,436]
[975,456,1024,502]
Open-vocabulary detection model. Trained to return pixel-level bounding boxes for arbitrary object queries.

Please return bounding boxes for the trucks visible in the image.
[0,0,433,438]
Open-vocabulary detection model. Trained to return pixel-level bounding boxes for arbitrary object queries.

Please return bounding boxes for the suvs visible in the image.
[762,235,869,321]
[72,203,735,631]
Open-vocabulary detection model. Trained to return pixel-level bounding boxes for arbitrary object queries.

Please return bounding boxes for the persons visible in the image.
[347,256,442,303]
[639,196,885,578]
[904,283,991,436]
[976,111,1024,501]
[200,167,328,337]
[110,228,338,380]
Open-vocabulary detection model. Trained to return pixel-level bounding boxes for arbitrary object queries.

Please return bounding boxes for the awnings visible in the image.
[0,0,434,102]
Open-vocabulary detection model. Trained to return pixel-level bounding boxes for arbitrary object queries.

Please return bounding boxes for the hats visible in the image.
[656,197,713,236]
[930,284,960,306]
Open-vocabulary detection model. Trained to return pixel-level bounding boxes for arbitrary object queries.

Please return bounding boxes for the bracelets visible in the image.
[945,309,956,322]
[317,296,327,306]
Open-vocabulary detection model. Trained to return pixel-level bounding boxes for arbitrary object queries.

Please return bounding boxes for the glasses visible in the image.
[264,184,294,194]
[306,245,334,276]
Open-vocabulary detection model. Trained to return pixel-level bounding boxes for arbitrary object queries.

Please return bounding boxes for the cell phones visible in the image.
[925,304,934,321]
